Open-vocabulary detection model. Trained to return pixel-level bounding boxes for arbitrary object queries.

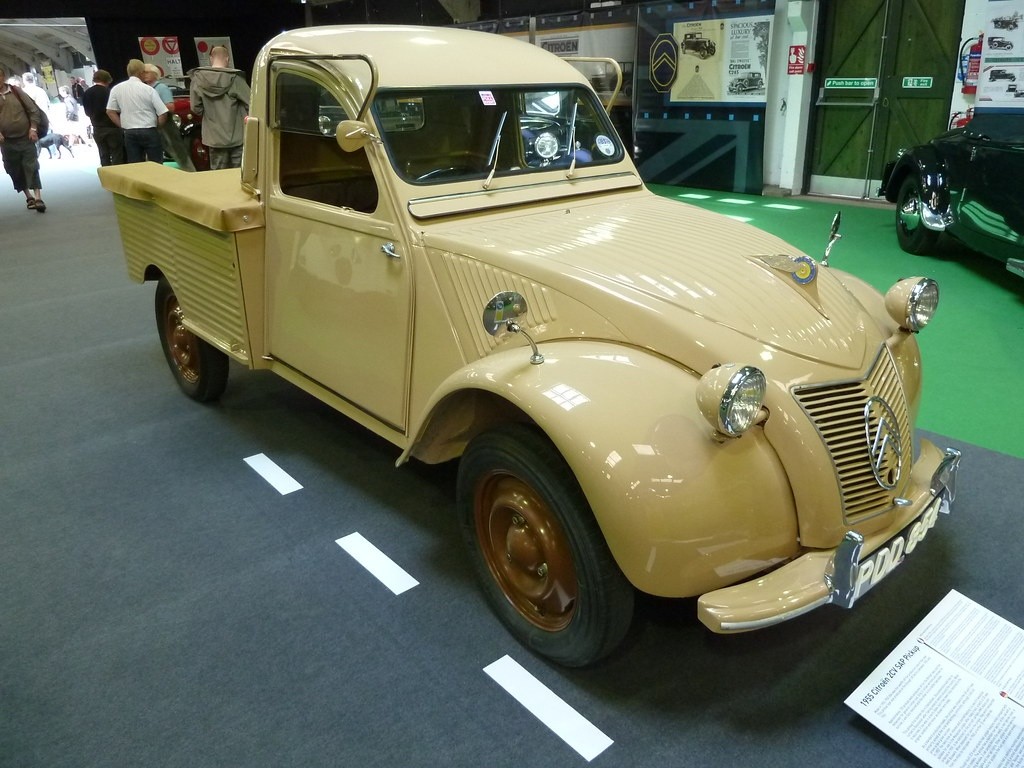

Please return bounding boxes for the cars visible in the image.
[170,89,593,170]
[728,72,764,92]
[681,32,716,60]
[989,70,1016,82]
[988,37,1013,51]
[875,114,1024,280]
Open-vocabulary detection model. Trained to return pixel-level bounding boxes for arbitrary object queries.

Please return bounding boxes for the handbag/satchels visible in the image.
[11,85,49,139]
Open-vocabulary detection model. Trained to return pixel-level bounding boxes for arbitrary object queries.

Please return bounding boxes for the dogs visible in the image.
[36,133,74,159]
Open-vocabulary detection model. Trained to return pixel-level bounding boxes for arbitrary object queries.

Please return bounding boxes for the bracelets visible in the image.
[31,128,37,132]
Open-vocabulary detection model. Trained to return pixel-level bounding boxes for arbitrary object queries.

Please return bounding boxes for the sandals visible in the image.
[35,199,45,209]
[27,198,35,209]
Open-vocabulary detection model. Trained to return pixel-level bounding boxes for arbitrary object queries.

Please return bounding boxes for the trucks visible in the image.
[97,27,962,670]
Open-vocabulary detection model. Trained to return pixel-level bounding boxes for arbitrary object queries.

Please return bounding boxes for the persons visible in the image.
[8,74,23,88]
[59,86,87,146]
[82,70,126,166]
[70,76,91,106]
[21,72,50,115]
[187,47,251,170]
[106,59,169,165]
[0,69,46,212]
[144,64,197,172]
